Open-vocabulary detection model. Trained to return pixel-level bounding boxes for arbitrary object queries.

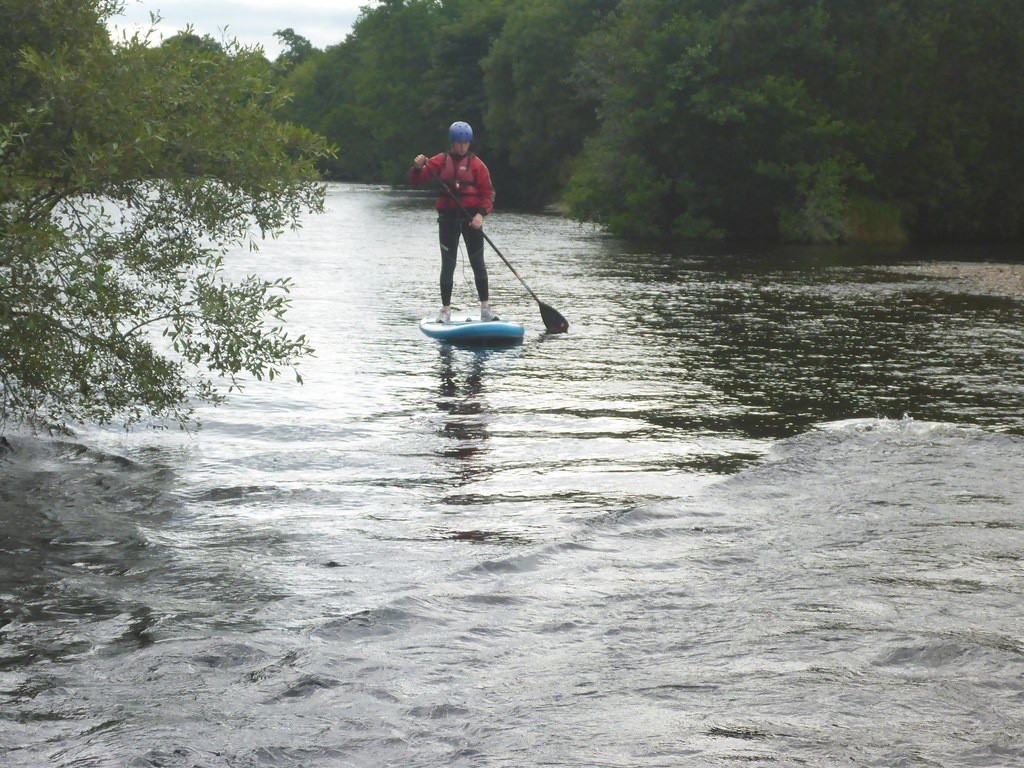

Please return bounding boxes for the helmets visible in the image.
[448,121,473,142]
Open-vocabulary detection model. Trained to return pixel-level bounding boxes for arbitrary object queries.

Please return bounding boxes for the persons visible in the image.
[409,121,501,323]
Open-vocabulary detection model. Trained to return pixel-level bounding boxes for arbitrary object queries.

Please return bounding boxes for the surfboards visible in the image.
[419,310,524,340]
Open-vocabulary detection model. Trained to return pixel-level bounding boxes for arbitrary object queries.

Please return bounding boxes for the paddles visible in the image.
[423,161,571,335]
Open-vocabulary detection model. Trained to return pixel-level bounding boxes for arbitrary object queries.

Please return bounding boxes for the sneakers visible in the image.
[481,309,499,322]
[436,308,450,323]
